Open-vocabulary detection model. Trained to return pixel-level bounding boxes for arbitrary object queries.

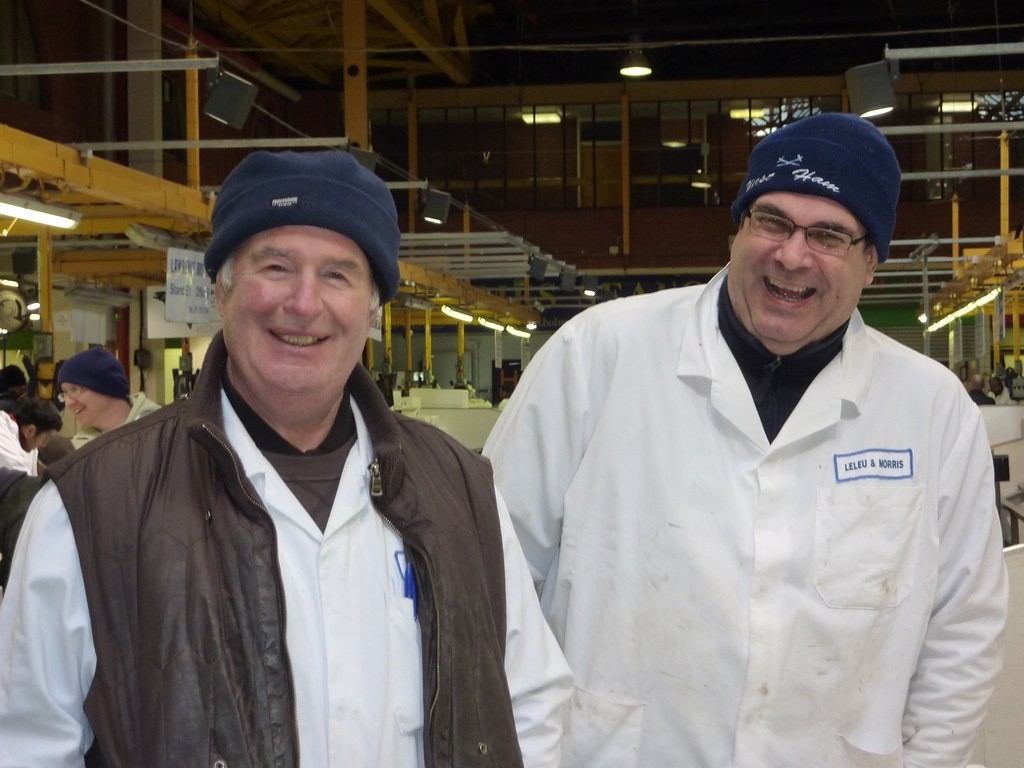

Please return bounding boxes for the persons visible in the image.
[481,114,1008,768]
[960,365,1018,509]
[4,152,574,768]
[0,347,162,603]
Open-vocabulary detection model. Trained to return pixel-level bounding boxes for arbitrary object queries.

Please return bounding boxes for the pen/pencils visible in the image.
[403,562,419,623]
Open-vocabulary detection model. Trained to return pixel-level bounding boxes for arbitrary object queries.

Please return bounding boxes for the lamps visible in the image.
[926,286,1002,332]
[620,46,652,77]
[0,51,258,128]
[67,136,377,173]
[27,298,41,309]
[0,191,83,230]
[398,232,607,312]
[506,324,531,339]
[124,221,212,253]
[202,181,453,225]
[441,304,473,322]
[64,281,133,307]
[397,291,435,312]
[845,42,1024,119]
[522,111,561,125]
[478,315,506,332]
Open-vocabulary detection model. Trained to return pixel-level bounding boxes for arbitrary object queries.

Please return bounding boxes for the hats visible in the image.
[57,348,130,397]
[205,151,400,305]
[731,113,901,263]
[0,365,26,390]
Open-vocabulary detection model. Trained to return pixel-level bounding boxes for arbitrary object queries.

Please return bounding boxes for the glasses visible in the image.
[748,209,868,258]
[57,385,86,403]
